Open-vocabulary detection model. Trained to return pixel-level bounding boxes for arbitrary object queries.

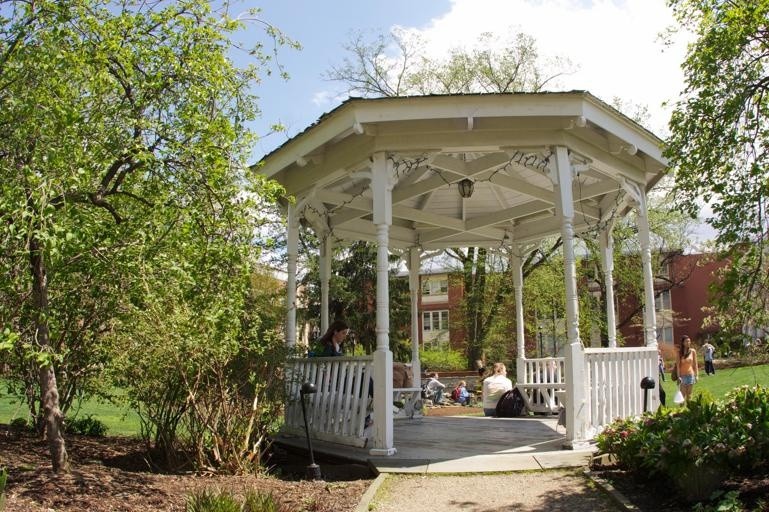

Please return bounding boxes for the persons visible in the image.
[483,362,513,417]
[676,335,698,408]
[426,372,445,404]
[658,348,667,381]
[313,320,373,430]
[701,339,715,377]
[457,380,469,406]
[533,357,560,415]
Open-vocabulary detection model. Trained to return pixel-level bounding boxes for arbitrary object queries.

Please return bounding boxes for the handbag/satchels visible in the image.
[671,363,677,381]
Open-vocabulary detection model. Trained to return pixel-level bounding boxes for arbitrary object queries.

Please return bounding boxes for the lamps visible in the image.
[458,178,474,198]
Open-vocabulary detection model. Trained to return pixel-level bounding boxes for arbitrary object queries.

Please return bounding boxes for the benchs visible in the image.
[393,386,423,417]
[302,387,374,444]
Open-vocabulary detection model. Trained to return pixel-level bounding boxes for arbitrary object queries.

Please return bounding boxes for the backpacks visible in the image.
[496,387,524,417]
[422,380,434,398]
[451,386,461,400]
